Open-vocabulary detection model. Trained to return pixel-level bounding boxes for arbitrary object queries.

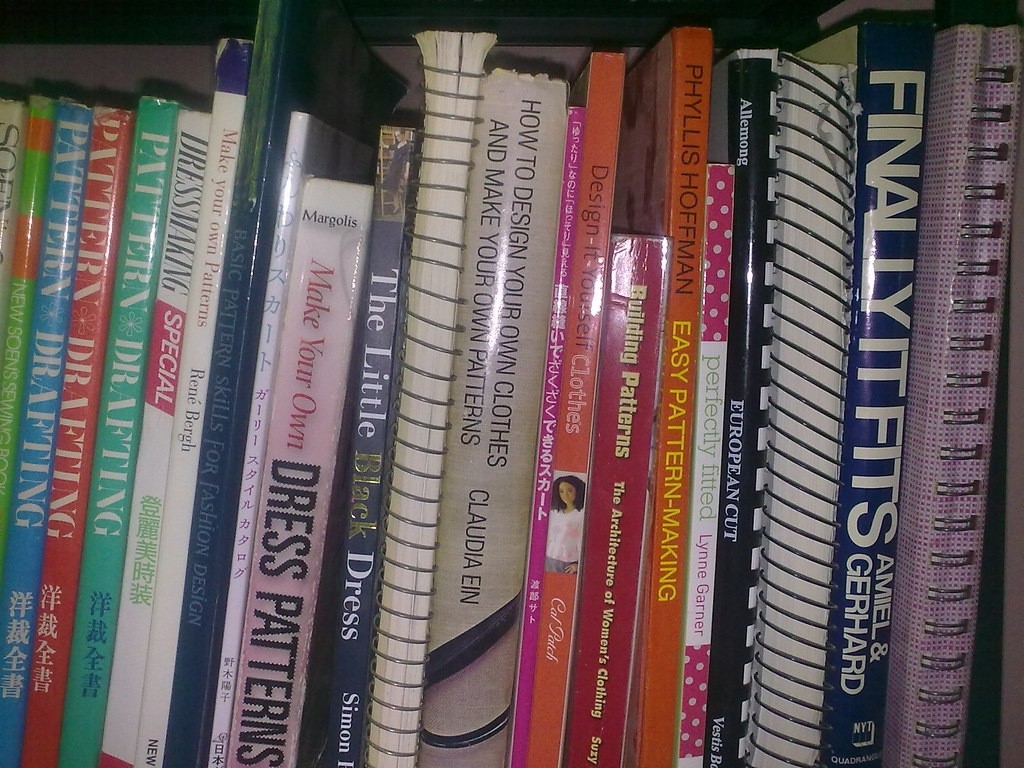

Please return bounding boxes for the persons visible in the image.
[544,475,587,575]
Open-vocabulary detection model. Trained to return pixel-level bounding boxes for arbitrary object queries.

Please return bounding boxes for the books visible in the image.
[0,10,1024,768]
